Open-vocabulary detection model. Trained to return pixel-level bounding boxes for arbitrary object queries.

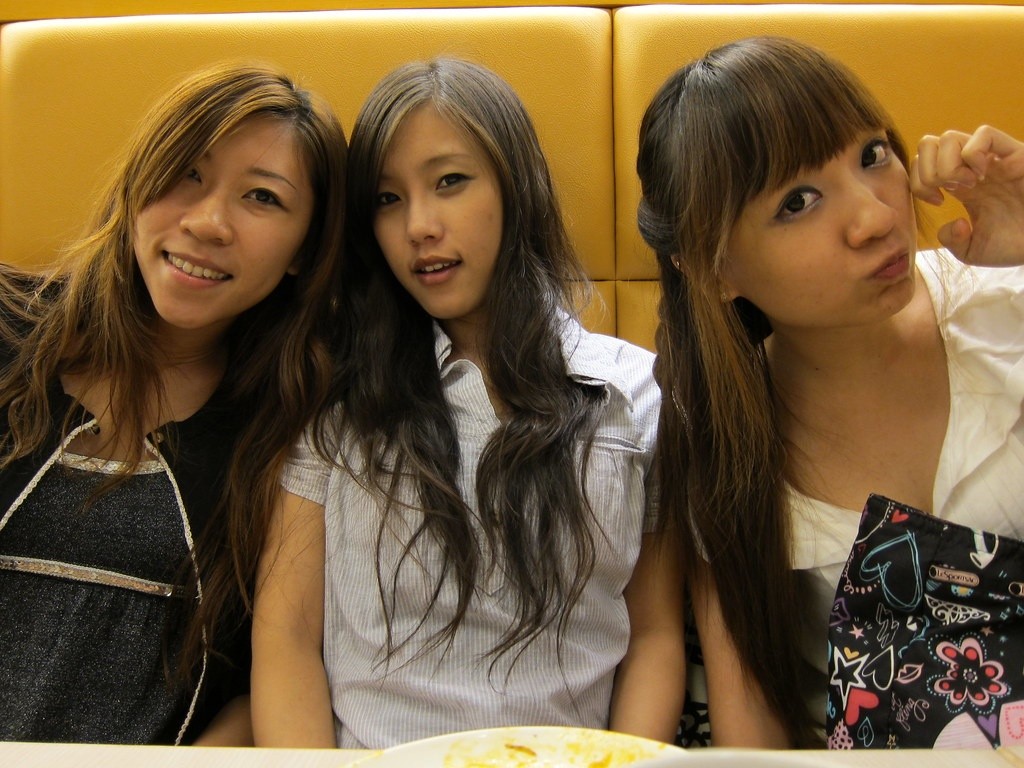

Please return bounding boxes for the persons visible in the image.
[0,62,350,753]
[633,32,1024,747]
[245,53,687,752]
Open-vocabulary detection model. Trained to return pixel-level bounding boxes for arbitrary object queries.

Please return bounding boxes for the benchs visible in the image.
[0,0,1024,352]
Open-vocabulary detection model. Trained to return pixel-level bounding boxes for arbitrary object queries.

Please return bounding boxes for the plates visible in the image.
[354,726,685,767]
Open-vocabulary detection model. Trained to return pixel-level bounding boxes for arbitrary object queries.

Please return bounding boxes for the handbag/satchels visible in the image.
[826,492,1024,749]
